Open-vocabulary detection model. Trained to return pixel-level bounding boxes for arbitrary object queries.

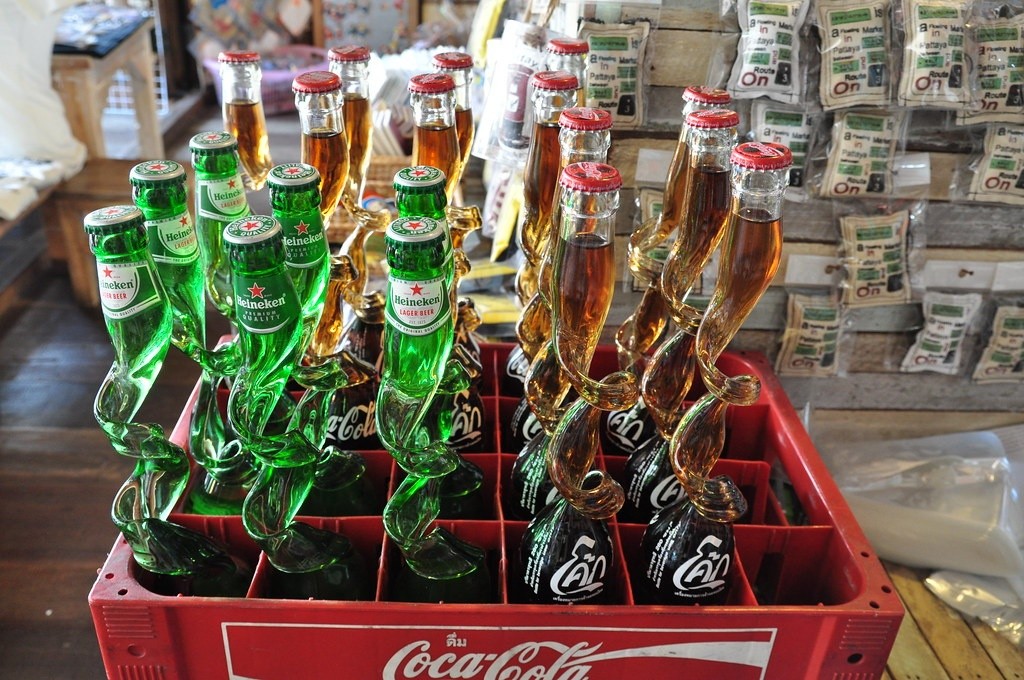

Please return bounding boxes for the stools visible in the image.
[50,6,165,163]
[54,156,194,311]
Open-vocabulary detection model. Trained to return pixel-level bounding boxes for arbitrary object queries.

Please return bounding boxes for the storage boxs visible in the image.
[87,334,906,680]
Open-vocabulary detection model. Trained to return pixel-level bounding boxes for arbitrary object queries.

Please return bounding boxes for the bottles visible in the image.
[84,36,791,605]
